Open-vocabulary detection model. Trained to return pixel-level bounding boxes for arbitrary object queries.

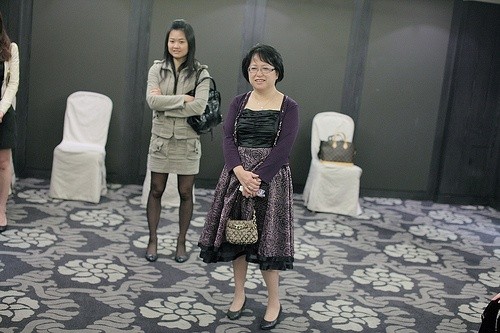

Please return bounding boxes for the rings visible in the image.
[247,185,250,189]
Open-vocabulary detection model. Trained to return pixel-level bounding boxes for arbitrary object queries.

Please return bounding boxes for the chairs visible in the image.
[48,91,113,203]
[302,111,362,216]
[141,155,195,207]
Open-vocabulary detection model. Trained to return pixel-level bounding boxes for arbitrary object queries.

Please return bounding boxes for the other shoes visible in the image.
[145,251,158,262]
[0,225,7,232]
[175,255,187,263]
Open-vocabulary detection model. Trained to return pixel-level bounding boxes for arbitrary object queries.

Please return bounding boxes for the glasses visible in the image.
[248,66,275,74]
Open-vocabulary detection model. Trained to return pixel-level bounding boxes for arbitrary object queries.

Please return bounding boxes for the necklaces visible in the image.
[254,90,278,109]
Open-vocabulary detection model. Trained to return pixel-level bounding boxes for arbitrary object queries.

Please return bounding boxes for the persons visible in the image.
[145,19,211,263]
[0,12,20,232]
[197,44,299,330]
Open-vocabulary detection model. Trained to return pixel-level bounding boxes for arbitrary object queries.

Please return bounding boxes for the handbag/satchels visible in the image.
[186,67,222,135]
[226,192,258,244]
[318,133,356,163]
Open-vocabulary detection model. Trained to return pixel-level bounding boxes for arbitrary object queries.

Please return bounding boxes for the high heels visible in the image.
[227,296,247,320]
[260,304,282,330]
